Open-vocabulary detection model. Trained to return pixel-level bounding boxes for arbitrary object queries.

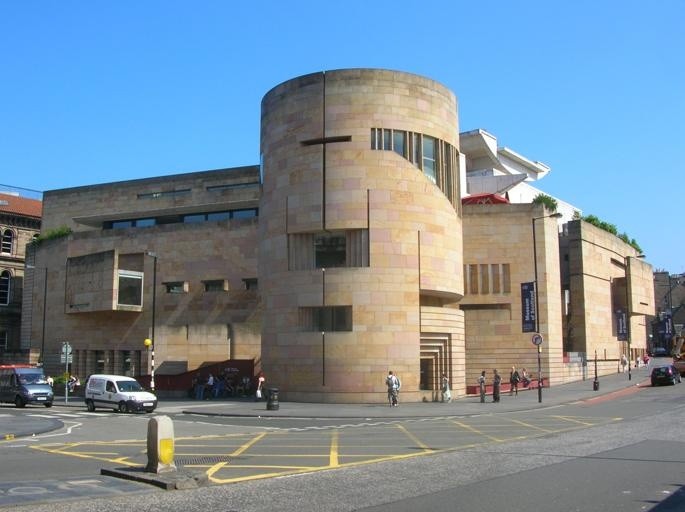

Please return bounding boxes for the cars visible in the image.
[651,365,682,386]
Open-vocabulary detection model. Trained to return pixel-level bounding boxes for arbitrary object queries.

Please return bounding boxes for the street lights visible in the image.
[314,267,326,385]
[142,249,157,388]
[23,264,47,362]
[532,213,564,402]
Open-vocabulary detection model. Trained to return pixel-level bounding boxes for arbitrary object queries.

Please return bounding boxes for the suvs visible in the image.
[84,373,157,413]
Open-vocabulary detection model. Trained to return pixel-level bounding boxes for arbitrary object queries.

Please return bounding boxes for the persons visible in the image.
[492,368,503,403]
[508,365,519,396]
[440,373,453,403]
[192,370,251,401]
[109,385,116,392]
[392,371,401,406]
[254,371,266,401]
[477,370,486,403]
[47,375,55,400]
[20,375,28,384]
[68,374,79,392]
[385,370,399,407]
[34,375,44,384]
[621,353,649,373]
[518,367,531,388]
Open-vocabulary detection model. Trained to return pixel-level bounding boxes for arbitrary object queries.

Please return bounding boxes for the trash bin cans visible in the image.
[267,388,280,411]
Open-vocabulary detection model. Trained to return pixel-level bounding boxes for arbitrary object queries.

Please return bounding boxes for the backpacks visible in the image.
[387,376,394,387]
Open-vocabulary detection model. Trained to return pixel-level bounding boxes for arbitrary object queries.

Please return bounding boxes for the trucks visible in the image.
[0,365,53,407]
